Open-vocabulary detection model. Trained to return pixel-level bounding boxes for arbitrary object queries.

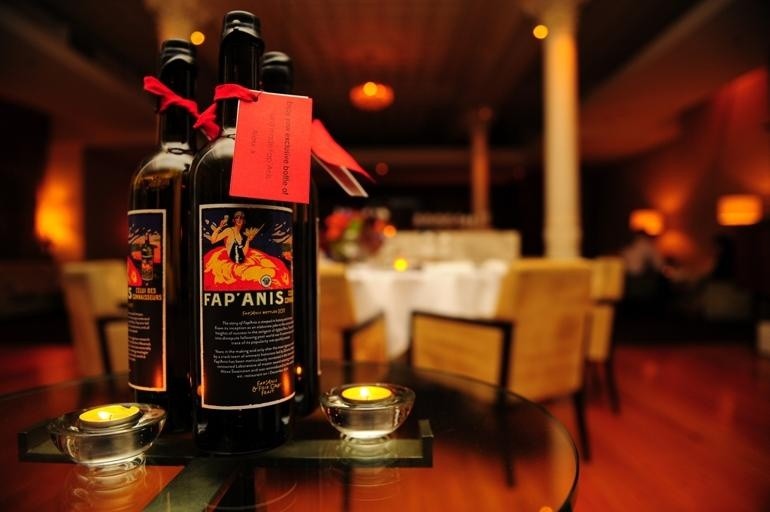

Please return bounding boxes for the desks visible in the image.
[0,360,580,512]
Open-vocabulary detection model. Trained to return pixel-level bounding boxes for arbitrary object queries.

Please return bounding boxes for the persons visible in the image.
[210,210,264,264]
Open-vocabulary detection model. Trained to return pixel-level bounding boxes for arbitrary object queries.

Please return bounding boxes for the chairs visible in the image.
[321,226,629,491]
[56,251,128,374]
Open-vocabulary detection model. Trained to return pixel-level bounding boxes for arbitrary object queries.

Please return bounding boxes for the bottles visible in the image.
[126,39,195,412]
[171,8,309,450]
[253,41,298,104]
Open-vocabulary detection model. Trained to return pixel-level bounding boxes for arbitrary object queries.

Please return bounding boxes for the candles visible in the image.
[45,401,170,467]
[319,378,417,454]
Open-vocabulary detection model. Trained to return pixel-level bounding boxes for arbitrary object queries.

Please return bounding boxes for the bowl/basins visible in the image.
[322,382,415,450]
[47,404,167,480]
